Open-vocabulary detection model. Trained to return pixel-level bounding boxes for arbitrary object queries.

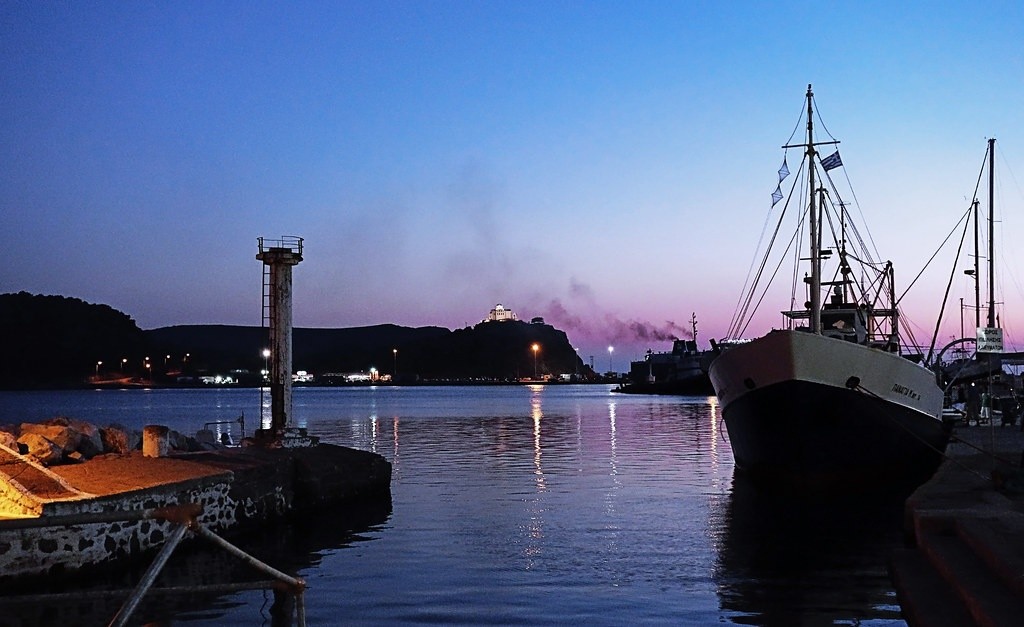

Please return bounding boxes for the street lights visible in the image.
[95,352,191,373]
[255,334,623,386]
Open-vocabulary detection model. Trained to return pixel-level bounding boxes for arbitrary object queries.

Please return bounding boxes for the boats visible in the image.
[709,81,949,516]
[905,138,1023,428]
[610,310,719,396]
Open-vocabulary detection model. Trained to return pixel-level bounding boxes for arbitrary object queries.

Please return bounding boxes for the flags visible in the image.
[818,149,843,173]
